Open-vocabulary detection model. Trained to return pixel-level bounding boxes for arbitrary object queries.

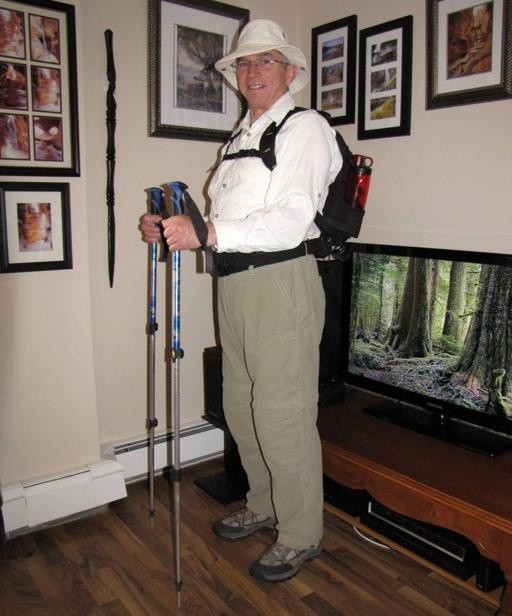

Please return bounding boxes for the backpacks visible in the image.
[211,105,375,284]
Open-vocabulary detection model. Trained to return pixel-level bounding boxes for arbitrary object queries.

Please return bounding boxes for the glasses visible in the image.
[230,57,288,73]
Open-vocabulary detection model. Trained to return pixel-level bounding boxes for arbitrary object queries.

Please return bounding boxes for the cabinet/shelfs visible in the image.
[190,347,511,616]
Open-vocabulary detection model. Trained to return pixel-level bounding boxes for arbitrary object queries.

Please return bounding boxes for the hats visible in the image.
[214,17,309,101]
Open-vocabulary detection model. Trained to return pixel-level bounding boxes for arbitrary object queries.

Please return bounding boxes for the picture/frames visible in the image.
[146,0,254,144]
[423,0,512,111]
[0,0,82,177]
[310,12,358,128]
[357,14,414,144]
[0,182,75,274]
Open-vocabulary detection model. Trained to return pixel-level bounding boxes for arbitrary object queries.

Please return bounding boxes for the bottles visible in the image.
[346,153,374,211]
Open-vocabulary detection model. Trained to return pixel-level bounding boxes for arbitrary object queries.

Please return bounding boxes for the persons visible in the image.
[138,17,342,582]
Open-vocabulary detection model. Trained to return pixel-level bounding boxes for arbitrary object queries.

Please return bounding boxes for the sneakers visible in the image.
[211,504,278,543]
[248,540,323,584]
